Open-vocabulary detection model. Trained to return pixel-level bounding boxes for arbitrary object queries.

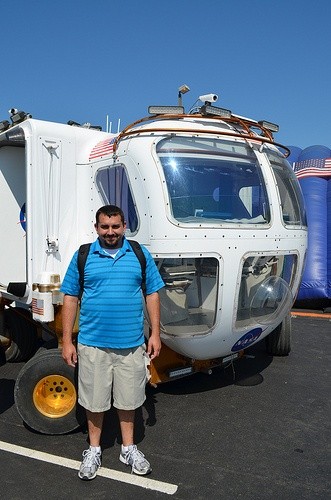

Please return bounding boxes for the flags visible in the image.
[89,137,121,160]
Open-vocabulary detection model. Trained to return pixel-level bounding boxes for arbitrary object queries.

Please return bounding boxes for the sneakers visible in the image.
[119,445,152,475]
[77,450,101,480]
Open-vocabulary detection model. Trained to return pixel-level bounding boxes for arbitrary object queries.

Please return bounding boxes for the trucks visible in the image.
[0,84,309,437]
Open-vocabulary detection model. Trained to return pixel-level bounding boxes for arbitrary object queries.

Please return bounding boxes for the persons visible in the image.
[60,205,165,480]
